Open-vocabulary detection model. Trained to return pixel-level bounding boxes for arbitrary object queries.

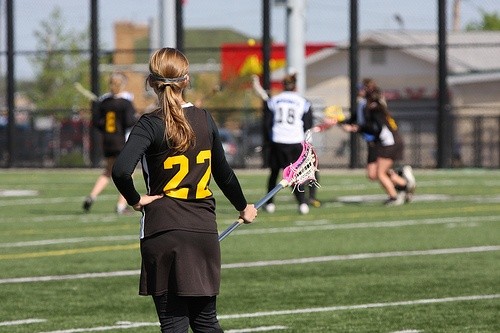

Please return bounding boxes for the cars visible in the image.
[217,126,246,168]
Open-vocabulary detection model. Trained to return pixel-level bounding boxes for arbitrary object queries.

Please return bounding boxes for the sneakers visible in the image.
[265,203,276,213]
[383,166,416,205]
[117,204,132,214]
[81,194,94,212]
[299,198,321,213]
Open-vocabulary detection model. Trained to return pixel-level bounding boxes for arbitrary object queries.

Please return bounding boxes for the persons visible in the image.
[326,79,412,203]
[252,73,321,207]
[263,75,313,214]
[83,80,141,214]
[351,85,416,205]
[111,47,258,333]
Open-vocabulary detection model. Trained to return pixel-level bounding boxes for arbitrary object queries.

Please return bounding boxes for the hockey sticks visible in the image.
[217,140,323,242]
[325,103,347,123]
[250,72,271,101]
[72,80,99,102]
